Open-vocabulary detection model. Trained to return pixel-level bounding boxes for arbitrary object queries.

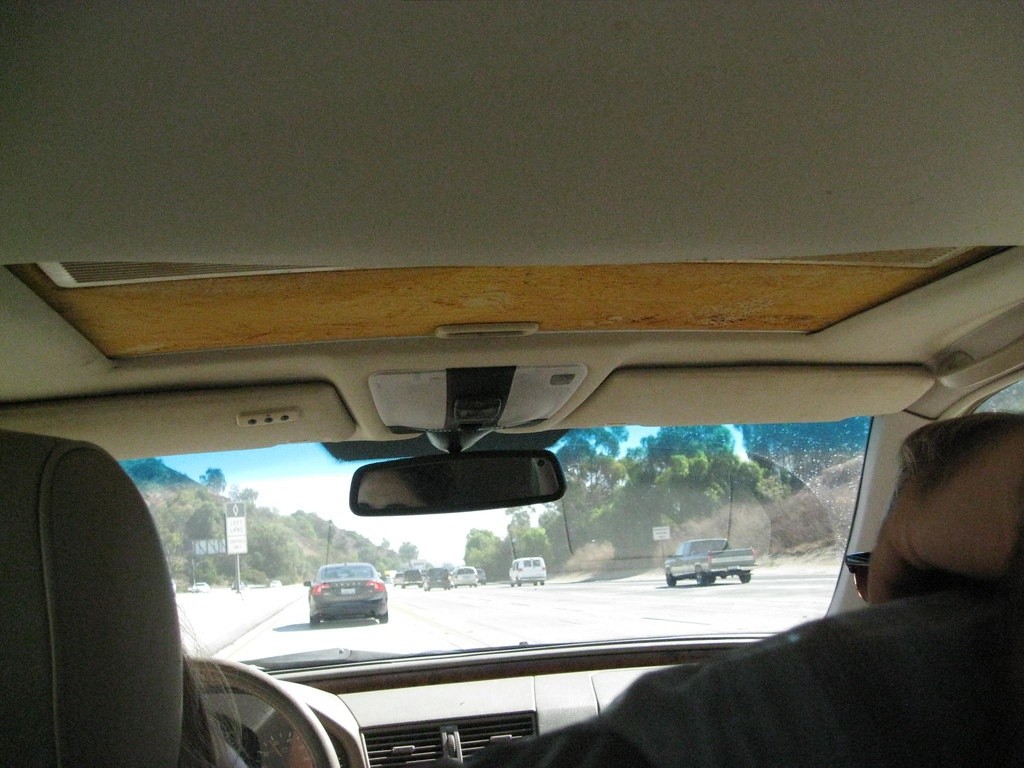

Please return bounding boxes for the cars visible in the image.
[232,580,245,591]
[270,580,282,588]
[304,562,388,623]
[191,582,210,593]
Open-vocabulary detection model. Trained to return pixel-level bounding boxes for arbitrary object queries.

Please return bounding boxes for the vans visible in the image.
[509,557,547,587]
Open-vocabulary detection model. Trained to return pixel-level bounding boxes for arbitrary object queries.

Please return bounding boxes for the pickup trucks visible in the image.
[665,539,758,587]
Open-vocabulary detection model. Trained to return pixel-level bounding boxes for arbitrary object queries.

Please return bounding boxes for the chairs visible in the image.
[0,438,183,766]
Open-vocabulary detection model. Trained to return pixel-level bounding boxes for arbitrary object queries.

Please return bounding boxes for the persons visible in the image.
[179,657,246,768]
[358,463,449,510]
[868,409,1023,600]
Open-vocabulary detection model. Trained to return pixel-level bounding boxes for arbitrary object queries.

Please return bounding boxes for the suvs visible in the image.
[393,567,486,591]
